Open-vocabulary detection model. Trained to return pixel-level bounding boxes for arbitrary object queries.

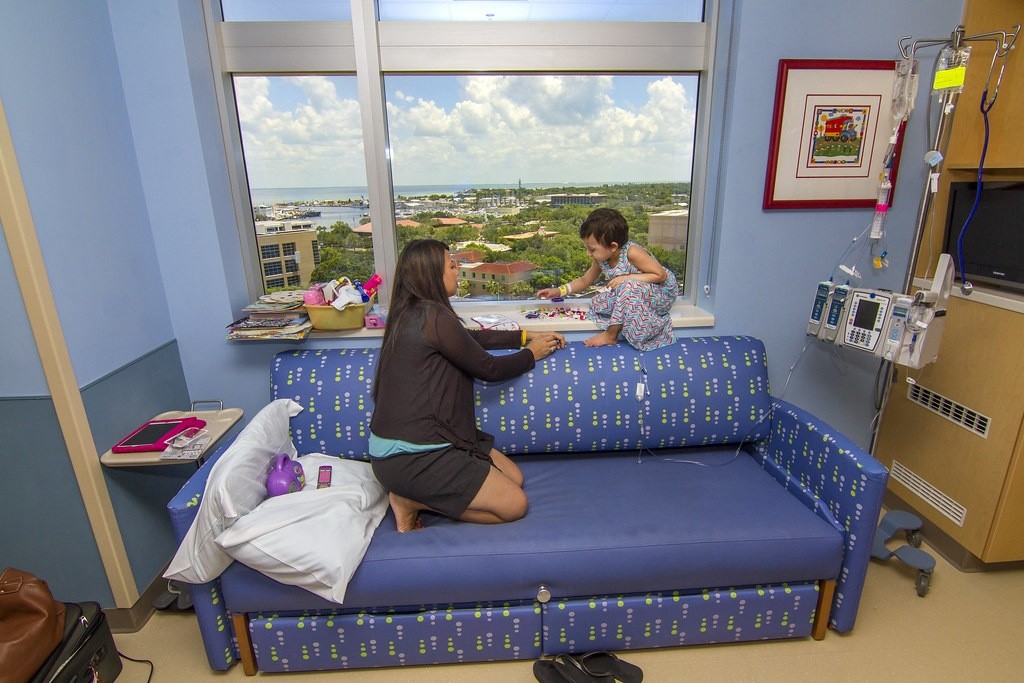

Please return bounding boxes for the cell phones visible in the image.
[317,466,332,489]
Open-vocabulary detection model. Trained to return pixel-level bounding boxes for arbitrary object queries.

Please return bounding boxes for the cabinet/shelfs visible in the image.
[868,0,1024,570]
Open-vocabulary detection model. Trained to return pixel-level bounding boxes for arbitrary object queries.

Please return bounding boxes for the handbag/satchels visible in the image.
[0,567,66,683]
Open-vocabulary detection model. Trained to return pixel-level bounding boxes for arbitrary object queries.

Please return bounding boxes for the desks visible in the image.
[100,400,245,613]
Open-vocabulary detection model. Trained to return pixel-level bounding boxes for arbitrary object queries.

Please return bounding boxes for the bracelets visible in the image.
[522,329,527,345]
[565,283,572,294]
[558,284,566,296]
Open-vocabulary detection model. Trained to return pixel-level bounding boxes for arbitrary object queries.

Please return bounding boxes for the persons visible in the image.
[367,238,565,533]
[536,206,680,351]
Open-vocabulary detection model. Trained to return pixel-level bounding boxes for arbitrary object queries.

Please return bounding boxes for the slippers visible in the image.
[574,650,643,683]
[533,654,616,683]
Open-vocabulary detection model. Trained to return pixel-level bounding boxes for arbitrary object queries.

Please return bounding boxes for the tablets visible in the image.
[112,417,206,450]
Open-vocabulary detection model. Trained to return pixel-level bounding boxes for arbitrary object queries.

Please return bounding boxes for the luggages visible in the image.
[27,601,124,683]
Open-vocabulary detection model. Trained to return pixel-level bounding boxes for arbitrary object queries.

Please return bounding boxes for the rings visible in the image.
[550,346,553,352]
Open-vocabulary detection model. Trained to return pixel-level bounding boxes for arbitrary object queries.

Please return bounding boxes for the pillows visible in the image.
[162,398,305,585]
[215,453,389,605]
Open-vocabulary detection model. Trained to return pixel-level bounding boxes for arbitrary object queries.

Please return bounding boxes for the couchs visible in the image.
[165,335,889,676]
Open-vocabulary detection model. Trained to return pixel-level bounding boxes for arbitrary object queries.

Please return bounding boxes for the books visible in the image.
[226,291,314,341]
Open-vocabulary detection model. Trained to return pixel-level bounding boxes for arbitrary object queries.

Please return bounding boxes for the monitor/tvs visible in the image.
[941,180,1024,289]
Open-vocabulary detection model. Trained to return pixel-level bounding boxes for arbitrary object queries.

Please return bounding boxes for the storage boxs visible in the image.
[302,291,376,329]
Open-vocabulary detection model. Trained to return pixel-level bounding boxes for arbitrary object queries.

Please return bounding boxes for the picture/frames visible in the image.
[762,58,908,211]
